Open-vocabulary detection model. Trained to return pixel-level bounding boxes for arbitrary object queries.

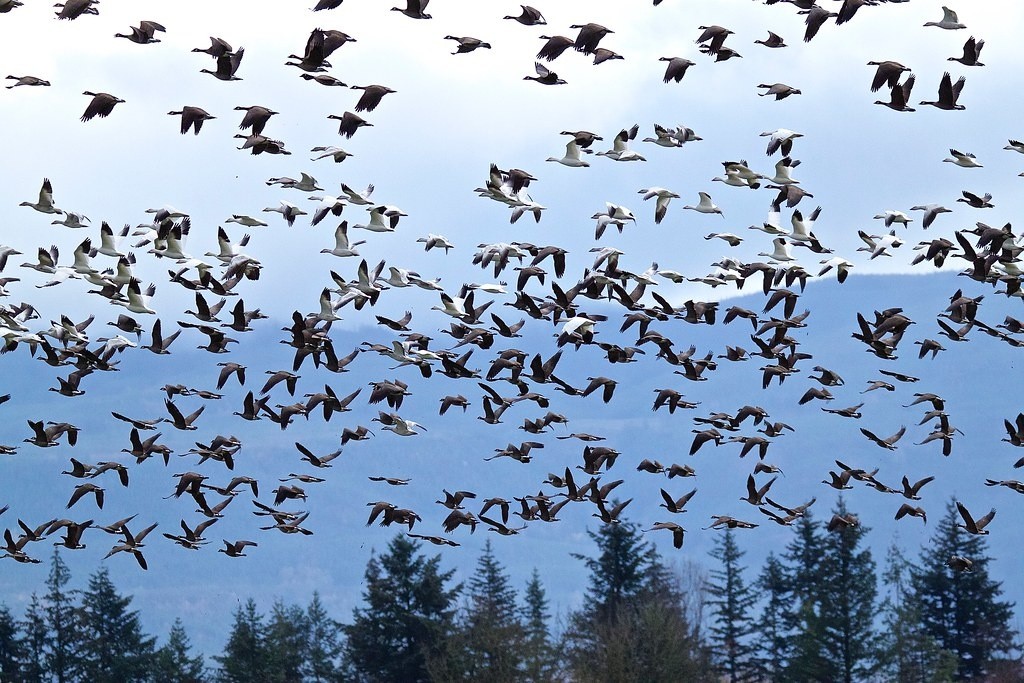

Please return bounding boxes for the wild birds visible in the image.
[0,0,1019,571]
